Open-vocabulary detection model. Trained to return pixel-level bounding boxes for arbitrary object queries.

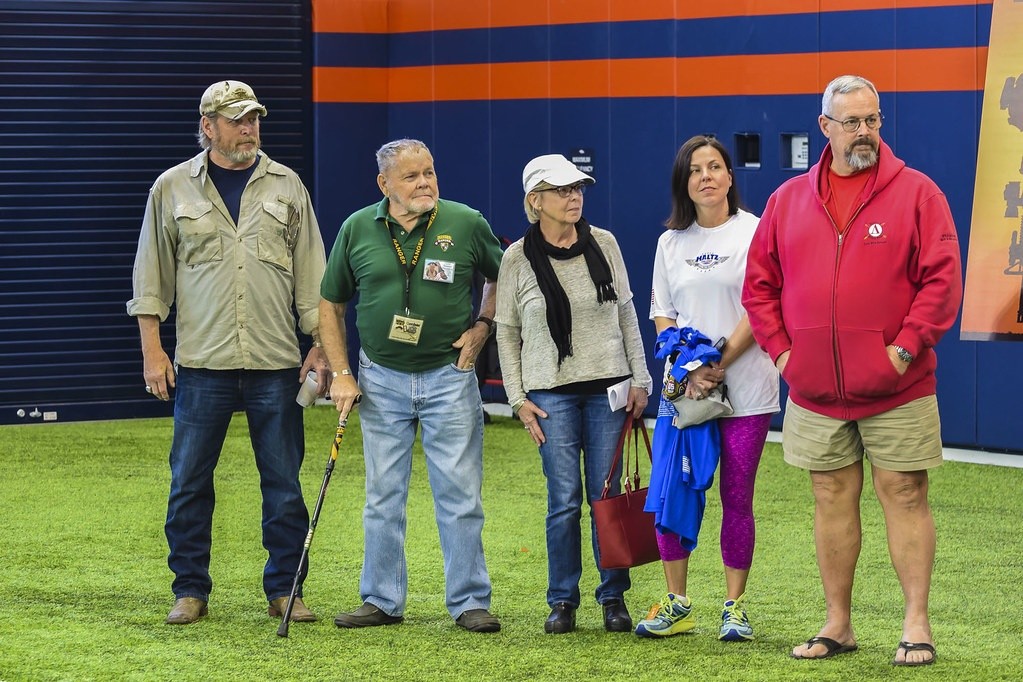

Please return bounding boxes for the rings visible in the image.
[145,386,152,393]
[525,426,530,429]
[469,362,474,366]
[696,392,701,397]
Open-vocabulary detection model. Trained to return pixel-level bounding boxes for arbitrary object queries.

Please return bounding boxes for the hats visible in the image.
[199,80,268,120]
[521,153,595,193]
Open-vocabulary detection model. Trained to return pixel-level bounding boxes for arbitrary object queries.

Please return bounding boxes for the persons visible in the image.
[496,152,652,637]
[316,139,509,634]
[635,136,781,641]
[426,263,437,277]
[125,80,328,626]
[740,72,963,667]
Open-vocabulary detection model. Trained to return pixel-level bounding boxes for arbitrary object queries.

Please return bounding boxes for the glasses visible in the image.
[824,116,884,133]
[532,181,587,197]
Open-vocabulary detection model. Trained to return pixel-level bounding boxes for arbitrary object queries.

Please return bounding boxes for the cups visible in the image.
[296,371,326,409]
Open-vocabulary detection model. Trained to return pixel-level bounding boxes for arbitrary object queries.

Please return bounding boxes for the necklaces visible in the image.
[826,171,872,224]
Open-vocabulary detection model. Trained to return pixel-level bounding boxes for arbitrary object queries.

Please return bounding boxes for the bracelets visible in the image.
[513,402,525,414]
[311,342,323,349]
[643,385,649,395]
[330,369,353,377]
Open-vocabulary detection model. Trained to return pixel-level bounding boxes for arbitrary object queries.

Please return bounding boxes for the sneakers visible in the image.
[635,593,696,637]
[602,599,632,630]
[545,602,576,634]
[269,596,315,621]
[456,609,501,631]
[166,596,208,623]
[718,591,754,640]
[335,603,403,628]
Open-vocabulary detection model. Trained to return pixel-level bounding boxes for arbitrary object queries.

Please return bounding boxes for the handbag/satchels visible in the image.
[594,411,661,569]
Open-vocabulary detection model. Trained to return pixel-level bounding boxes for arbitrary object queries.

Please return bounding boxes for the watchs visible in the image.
[896,345,913,364]
[471,317,496,337]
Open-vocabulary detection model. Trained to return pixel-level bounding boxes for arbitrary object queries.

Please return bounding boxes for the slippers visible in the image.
[894,642,935,665]
[793,637,858,660]
